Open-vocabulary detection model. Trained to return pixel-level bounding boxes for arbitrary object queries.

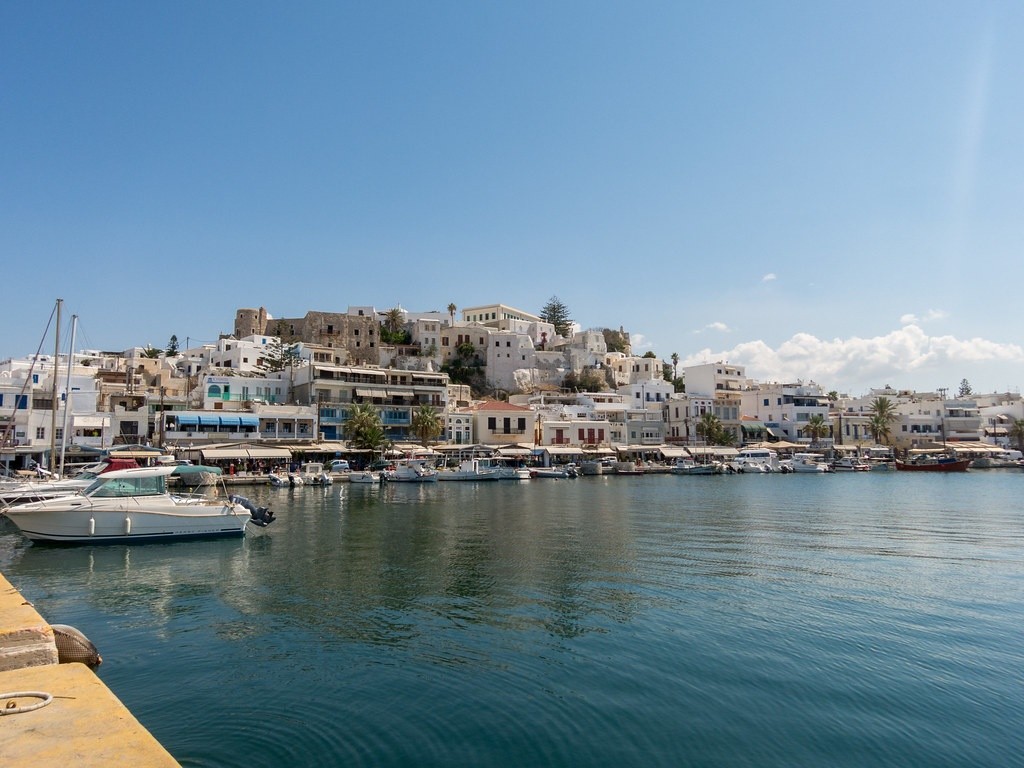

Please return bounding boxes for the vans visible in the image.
[997,451,1023,461]
[734,450,771,465]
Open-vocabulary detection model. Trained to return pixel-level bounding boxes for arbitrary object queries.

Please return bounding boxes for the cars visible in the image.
[364,460,388,472]
[326,459,349,472]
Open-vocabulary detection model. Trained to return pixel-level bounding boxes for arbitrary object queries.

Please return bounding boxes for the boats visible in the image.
[0,458,252,546]
[267,457,871,486]
[896,448,970,471]
